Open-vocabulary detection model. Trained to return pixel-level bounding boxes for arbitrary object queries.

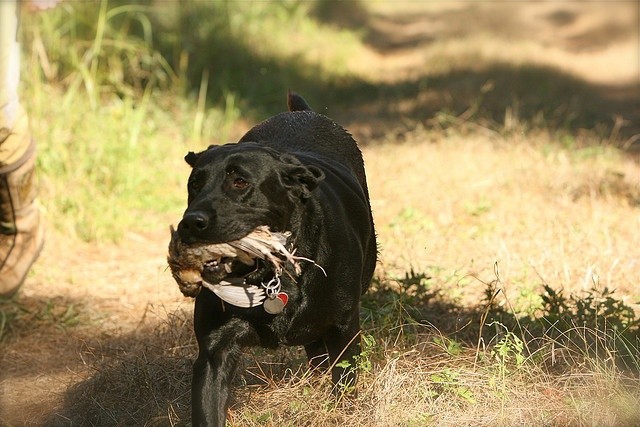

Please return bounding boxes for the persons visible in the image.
[1,0,61,299]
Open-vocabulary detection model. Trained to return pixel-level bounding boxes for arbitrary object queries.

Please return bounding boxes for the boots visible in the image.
[0,139,46,299]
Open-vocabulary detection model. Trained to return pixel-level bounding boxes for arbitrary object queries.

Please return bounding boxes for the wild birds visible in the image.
[167,224,303,309]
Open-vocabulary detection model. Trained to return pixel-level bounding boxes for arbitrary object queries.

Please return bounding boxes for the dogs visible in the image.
[177,87,377,427]
